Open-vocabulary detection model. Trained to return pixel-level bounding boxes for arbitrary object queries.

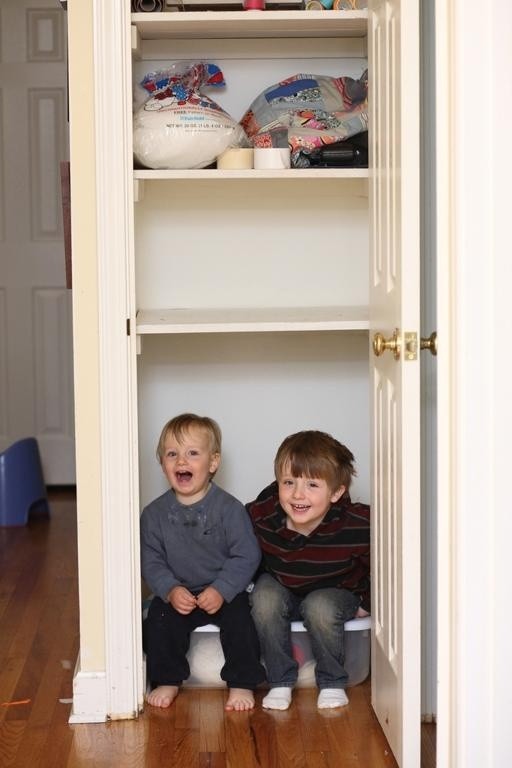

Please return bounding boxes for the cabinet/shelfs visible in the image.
[106,0,372,728]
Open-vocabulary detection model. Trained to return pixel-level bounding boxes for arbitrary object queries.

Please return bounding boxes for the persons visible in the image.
[244,429,371,711]
[138,412,265,713]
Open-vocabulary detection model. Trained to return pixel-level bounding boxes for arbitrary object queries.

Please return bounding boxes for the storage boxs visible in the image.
[180,615,371,693]
